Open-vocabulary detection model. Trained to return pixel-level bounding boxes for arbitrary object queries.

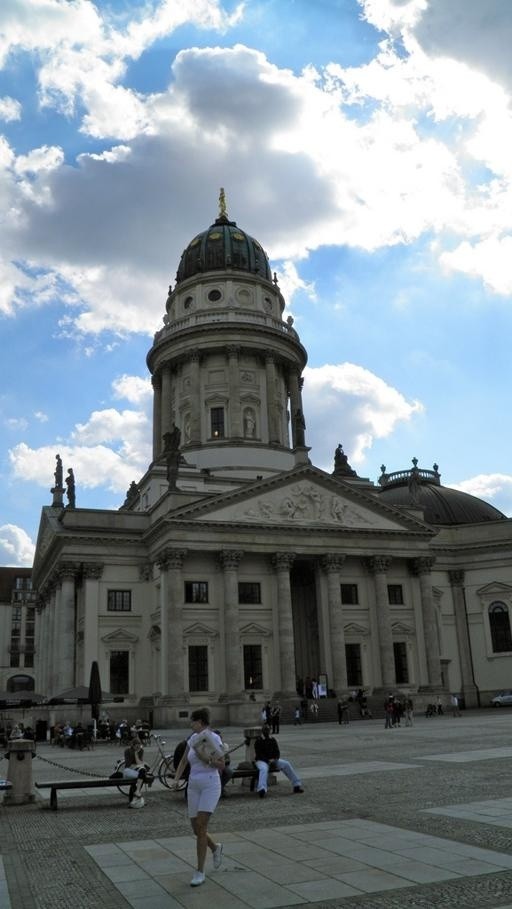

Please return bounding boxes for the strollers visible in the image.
[422,704,437,718]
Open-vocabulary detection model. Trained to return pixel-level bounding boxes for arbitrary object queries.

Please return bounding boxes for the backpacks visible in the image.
[387,703,394,712]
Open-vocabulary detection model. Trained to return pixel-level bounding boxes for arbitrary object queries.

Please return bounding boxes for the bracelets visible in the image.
[207,757,213,767]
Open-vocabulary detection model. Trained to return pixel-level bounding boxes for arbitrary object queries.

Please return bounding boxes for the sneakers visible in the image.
[384,724,413,729]
[258,788,265,798]
[275,732,279,734]
[293,786,304,793]
[272,732,275,734]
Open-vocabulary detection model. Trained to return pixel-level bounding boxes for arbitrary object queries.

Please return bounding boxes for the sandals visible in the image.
[133,792,142,798]
[127,803,133,809]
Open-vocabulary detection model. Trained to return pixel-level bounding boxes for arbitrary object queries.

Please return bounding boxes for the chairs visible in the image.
[119,727,153,747]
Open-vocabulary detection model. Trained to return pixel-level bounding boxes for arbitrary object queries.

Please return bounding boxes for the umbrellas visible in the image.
[89,660,101,739]
[52,686,112,719]
[0,690,46,719]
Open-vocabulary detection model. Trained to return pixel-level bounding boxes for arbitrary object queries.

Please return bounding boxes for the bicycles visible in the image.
[163,758,187,791]
[115,733,186,797]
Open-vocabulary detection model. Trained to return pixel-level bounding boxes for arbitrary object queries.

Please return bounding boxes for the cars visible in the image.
[490,689,512,707]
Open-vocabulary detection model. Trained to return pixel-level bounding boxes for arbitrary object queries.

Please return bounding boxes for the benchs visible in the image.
[35,776,137,807]
[223,769,279,792]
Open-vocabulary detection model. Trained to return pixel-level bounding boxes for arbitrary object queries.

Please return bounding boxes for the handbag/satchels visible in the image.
[260,710,267,723]
[123,767,140,780]
[129,791,145,809]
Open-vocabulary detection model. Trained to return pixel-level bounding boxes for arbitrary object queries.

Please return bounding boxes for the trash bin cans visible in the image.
[36,720,47,740]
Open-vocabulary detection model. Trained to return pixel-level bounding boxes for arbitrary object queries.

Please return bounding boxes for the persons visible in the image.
[257,484,345,521]
[66,469,75,506]
[336,444,343,456]
[214,730,232,797]
[173,707,225,886]
[124,739,146,808]
[54,456,62,487]
[255,723,304,798]
[7,719,152,748]
[245,411,254,434]
[173,736,189,797]
[249,677,461,734]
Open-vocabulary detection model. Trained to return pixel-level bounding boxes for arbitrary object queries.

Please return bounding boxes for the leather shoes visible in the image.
[190,870,206,887]
[211,842,224,869]
